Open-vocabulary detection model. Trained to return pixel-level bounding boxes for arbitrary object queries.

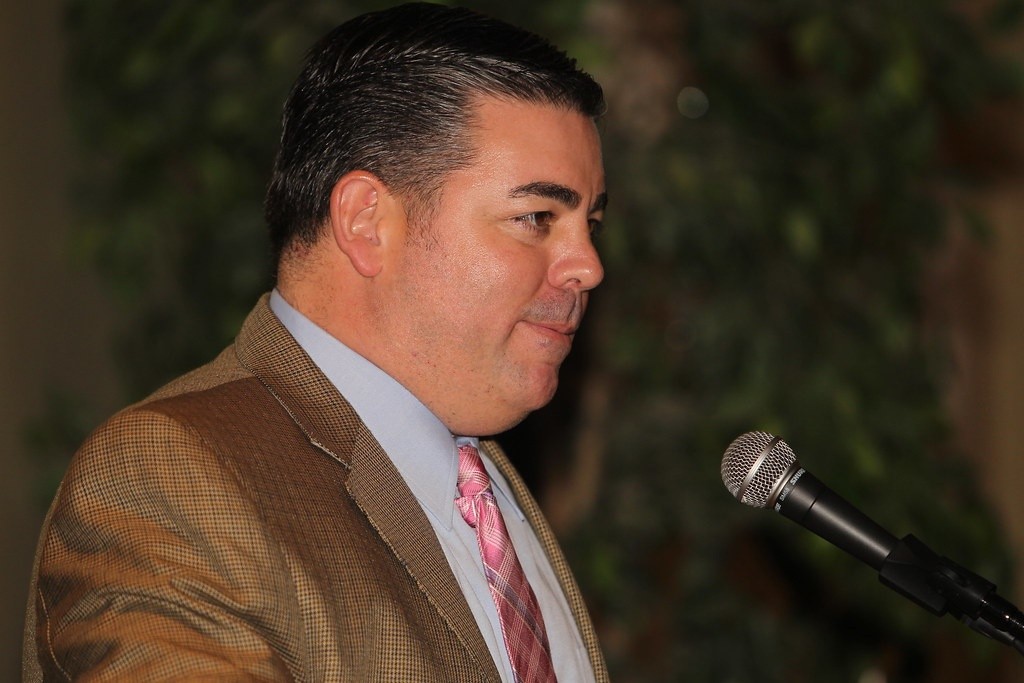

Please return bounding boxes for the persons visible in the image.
[21,2,614,683]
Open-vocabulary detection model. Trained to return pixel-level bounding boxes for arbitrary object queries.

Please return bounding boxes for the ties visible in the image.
[455,440,560,683]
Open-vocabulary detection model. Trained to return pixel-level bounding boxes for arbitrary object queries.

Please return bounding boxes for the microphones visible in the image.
[720,431,1023,657]
